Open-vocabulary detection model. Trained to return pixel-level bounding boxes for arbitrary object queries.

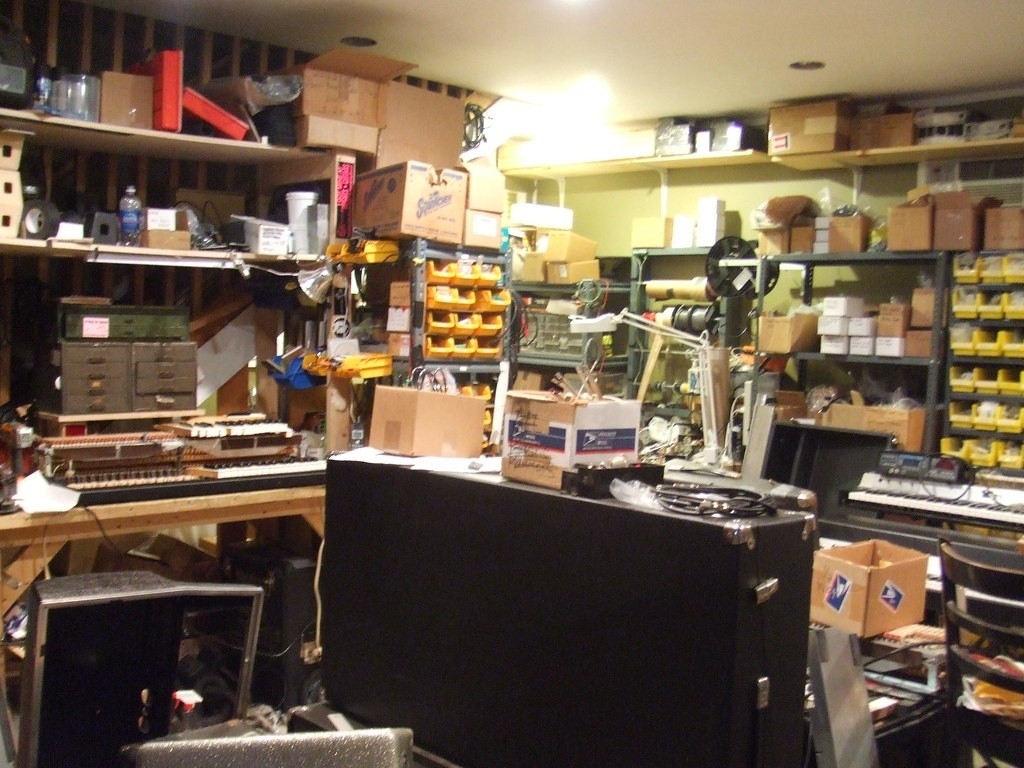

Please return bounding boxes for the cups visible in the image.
[59,72,101,124]
[286,191,319,255]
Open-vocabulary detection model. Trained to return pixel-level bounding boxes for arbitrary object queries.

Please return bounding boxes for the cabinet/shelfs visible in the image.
[0,106,1024,768]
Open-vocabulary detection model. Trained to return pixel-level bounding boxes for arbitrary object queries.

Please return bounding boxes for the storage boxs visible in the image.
[879,315,909,337]
[791,216,817,253]
[631,217,673,248]
[933,189,1004,251]
[875,337,905,357]
[759,312,821,355]
[820,335,849,355]
[905,331,944,357]
[525,253,549,284]
[462,208,502,250]
[294,115,377,158]
[546,259,600,285]
[347,160,472,249]
[369,384,485,458]
[138,229,191,250]
[848,317,878,336]
[887,185,934,251]
[544,230,599,262]
[503,390,641,493]
[817,316,847,337]
[825,296,863,318]
[97,70,154,130]
[829,216,871,253]
[229,213,292,255]
[295,47,419,131]
[849,337,874,356]
[910,288,948,327]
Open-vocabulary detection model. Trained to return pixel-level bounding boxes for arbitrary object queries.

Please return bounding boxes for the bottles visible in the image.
[118,185,142,247]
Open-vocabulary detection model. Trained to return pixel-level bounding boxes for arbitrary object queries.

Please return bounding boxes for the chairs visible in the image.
[935,531,1024,768]
